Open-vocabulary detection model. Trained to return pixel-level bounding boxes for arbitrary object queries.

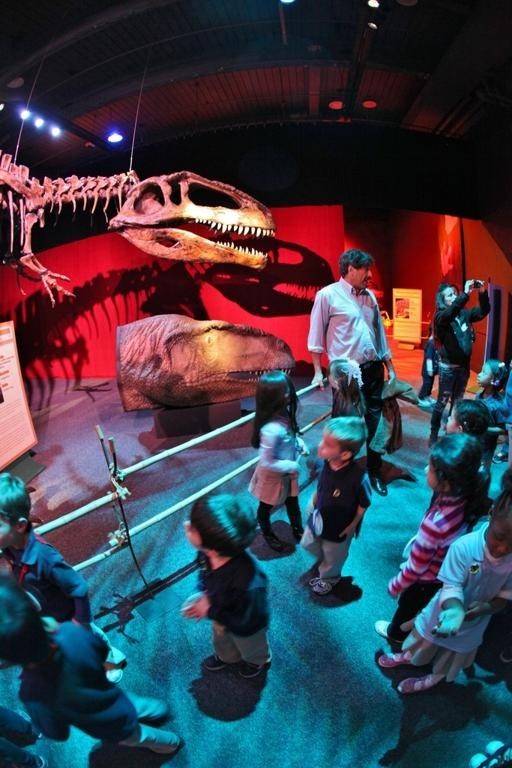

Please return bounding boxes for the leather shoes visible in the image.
[369,471,388,496]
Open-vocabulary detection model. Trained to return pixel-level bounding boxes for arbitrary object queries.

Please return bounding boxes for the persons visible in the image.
[304,246,399,497]
[0,704,50,768]
[375,359,512,698]
[416,319,439,409]
[469,740,511,767]
[299,416,370,596]
[327,358,365,419]
[1,571,187,762]
[176,488,277,681]
[426,278,492,450]
[0,472,129,684]
[249,371,310,554]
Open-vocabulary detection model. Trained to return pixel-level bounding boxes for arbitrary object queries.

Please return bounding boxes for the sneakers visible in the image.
[35,754,48,768]
[263,533,297,554]
[288,526,304,544]
[203,654,228,671]
[425,395,437,405]
[106,667,185,754]
[311,577,342,596]
[500,648,512,661]
[492,450,509,464]
[417,396,434,413]
[13,709,44,740]
[468,741,512,768]
[237,660,265,679]
[300,560,323,586]
[374,620,403,643]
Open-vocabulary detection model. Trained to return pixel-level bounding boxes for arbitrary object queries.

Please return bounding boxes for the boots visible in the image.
[428,408,442,448]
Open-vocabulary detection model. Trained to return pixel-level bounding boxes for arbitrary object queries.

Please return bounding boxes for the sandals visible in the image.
[378,651,413,667]
[397,674,431,693]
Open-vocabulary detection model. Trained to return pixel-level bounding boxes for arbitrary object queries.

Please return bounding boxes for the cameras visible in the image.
[474,280,484,288]
